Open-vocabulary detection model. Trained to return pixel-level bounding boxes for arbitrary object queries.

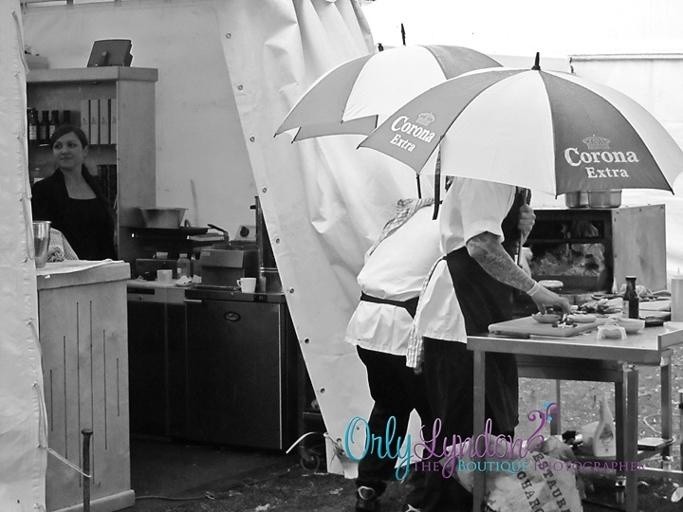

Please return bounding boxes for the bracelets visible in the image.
[526,280,541,297]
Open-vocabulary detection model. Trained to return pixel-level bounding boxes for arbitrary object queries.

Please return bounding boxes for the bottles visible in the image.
[177,254,190,279]
[623,276,638,319]
[28,110,71,144]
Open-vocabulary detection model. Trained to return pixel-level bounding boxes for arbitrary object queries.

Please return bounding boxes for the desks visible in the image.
[467,289,682,511]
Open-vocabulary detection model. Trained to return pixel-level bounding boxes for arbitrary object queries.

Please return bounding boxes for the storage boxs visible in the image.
[200,243,259,288]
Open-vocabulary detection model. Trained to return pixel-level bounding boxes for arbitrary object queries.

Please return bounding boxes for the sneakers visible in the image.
[355,485,378,512]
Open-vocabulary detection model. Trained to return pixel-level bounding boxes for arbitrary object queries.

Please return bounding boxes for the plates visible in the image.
[638,301,672,319]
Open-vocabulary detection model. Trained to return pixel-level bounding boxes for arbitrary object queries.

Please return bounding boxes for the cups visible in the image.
[237,278,257,293]
[157,270,173,284]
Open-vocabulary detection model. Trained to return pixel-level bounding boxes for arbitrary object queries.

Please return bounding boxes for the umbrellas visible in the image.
[357,52,683,268]
[292,44,423,198]
[274,24,502,137]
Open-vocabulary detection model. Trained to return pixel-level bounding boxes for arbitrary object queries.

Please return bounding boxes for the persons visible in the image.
[345,198,446,512]
[31,124,114,260]
[397,176,571,512]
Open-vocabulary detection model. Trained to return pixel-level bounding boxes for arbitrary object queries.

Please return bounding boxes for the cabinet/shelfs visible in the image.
[182,286,311,454]
[38,259,135,512]
[127,298,182,448]
[520,203,667,297]
[25,67,158,260]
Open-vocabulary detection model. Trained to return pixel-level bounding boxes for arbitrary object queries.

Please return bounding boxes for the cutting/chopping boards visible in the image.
[488,309,600,337]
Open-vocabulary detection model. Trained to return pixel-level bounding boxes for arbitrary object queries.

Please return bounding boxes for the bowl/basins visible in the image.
[565,192,588,208]
[588,191,622,208]
[618,318,645,333]
